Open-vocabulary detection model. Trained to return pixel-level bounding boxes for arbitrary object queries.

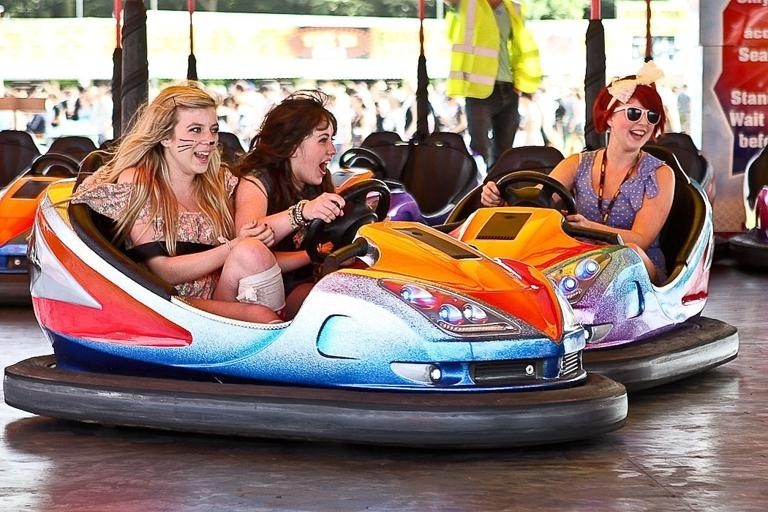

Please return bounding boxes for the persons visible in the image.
[69,81,289,327]
[479,73,677,286]
[439,0,547,172]
[1,77,696,168]
[234,93,393,323]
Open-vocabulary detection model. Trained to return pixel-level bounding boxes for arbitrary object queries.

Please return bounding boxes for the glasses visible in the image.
[613,106,662,125]
